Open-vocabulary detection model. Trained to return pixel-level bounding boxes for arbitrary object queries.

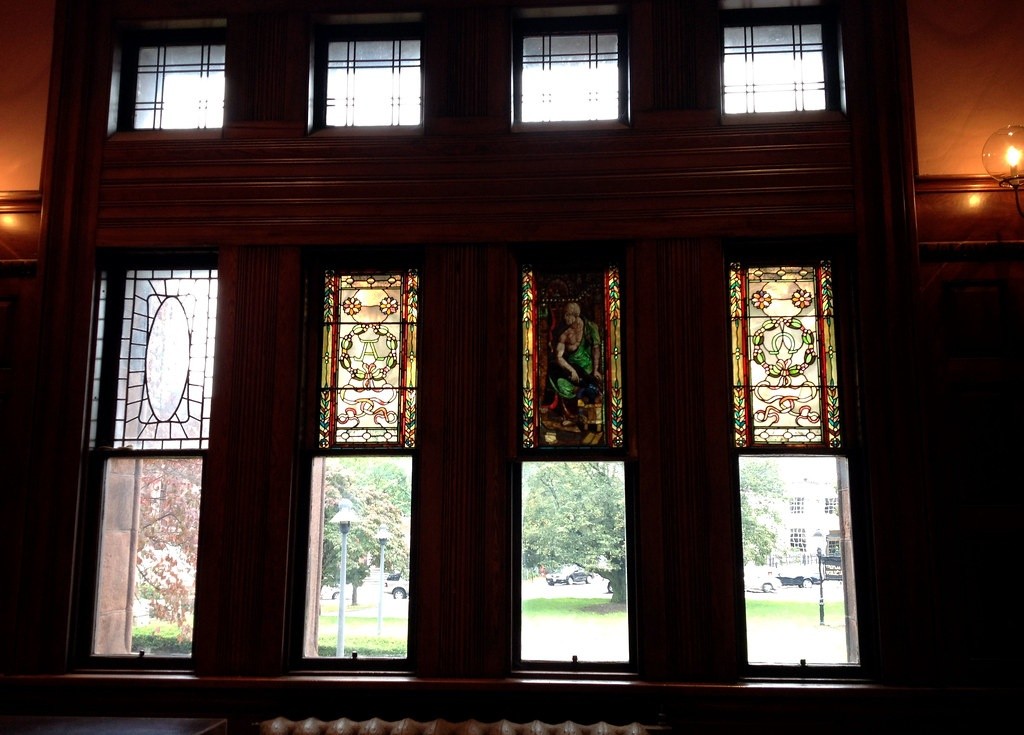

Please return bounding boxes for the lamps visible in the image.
[982,125,1024,218]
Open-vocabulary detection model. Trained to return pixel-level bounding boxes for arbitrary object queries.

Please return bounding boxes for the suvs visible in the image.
[384,571,410,600]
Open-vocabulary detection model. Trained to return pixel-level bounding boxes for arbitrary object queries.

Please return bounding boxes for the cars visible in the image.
[546,564,594,585]
[775,566,827,588]
[320,584,339,599]
[744,566,782,592]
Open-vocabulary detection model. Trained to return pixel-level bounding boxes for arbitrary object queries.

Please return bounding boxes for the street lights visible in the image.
[374,523,393,638]
[813,528,826,625]
[329,497,360,657]
